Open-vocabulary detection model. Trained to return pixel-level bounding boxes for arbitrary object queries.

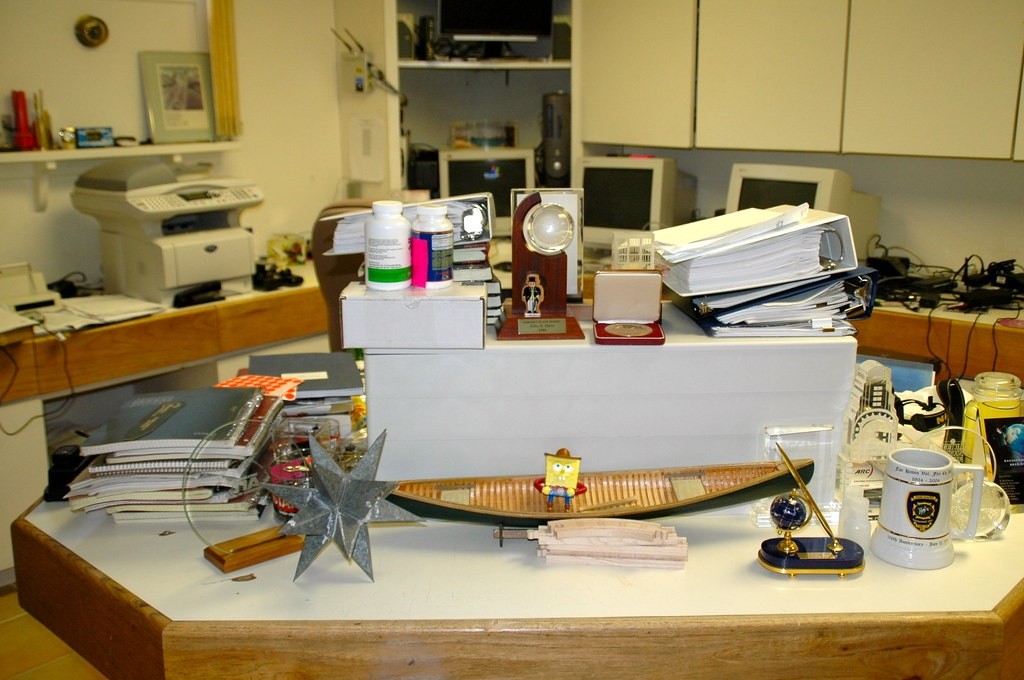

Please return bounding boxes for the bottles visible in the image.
[364,200,412,293]
[961,373,1024,512]
[409,204,456,291]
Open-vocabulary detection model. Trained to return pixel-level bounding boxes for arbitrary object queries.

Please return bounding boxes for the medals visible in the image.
[605,323,653,336]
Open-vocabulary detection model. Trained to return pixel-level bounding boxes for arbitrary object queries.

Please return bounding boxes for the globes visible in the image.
[770,489,813,554]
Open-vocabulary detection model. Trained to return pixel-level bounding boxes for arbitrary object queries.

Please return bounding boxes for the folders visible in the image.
[653,203,858,296]
[671,266,880,330]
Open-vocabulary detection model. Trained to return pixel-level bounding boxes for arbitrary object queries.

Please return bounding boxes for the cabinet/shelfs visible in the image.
[332,2,572,205]
[575,2,1024,157]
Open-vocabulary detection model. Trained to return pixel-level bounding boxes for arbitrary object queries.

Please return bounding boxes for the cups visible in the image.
[871,453,982,570]
[951,481,1011,539]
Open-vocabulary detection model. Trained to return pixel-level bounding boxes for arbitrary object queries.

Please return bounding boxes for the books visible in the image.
[64,353,364,524]
[452,243,503,324]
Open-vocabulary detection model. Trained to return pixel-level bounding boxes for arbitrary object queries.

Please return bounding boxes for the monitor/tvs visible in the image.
[726,165,882,266]
[570,154,698,265]
[438,0,553,59]
[439,147,535,236]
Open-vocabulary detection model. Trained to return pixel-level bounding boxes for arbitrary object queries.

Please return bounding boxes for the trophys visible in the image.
[497,191,585,340]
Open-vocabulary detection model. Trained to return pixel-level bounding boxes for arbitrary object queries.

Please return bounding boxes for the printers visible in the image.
[70,156,265,310]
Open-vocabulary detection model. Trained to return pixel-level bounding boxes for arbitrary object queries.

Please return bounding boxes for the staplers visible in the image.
[173,281,226,308]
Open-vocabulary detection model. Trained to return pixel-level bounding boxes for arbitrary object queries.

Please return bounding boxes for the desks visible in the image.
[0,252,1022,679]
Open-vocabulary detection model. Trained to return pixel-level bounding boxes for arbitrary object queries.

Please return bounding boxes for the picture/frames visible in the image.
[138,50,219,143]
